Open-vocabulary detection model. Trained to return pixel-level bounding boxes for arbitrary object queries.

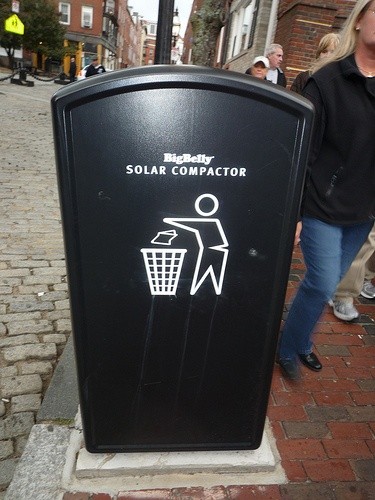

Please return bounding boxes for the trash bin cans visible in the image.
[50,64,316,454]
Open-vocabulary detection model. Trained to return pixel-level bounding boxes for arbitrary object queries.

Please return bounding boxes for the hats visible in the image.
[252,55,268,66]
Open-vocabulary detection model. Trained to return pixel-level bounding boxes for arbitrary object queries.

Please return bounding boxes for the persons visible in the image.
[290,33,342,95]
[275,0,375,383]
[70,55,76,83]
[252,56,270,79]
[85,56,106,78]
[245,44,287,88]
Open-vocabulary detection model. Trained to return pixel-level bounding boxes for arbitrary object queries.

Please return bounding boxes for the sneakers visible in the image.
[328,294,359,321]
[359,278,375,298]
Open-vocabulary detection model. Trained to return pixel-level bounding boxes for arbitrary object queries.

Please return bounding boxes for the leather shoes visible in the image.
[277,352,321,382]
